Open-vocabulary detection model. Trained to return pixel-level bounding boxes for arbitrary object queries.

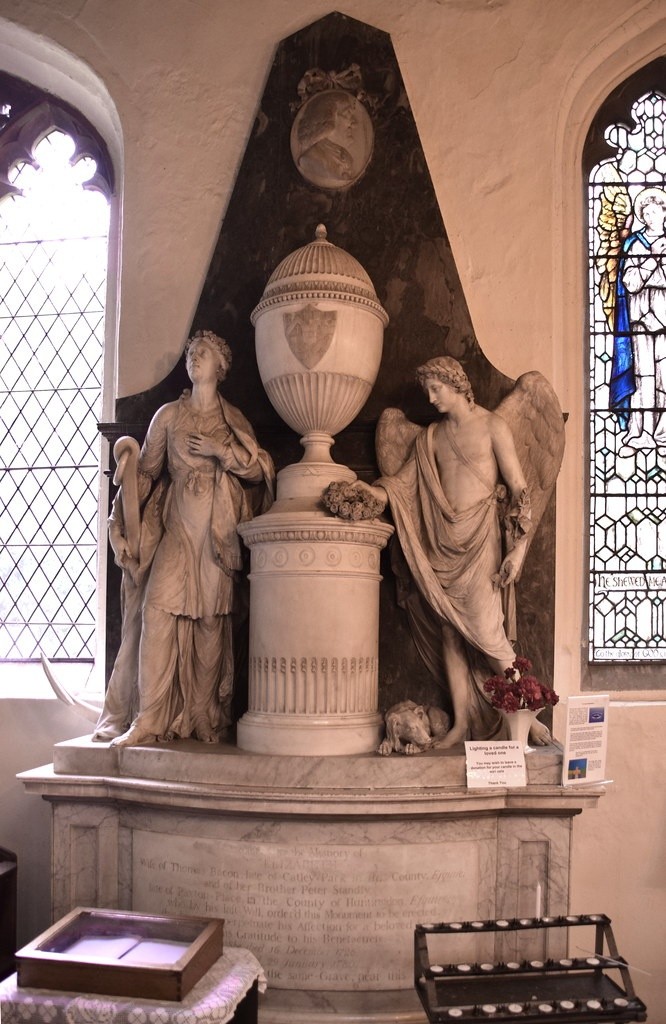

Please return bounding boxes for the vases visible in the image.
[494,706,546,754]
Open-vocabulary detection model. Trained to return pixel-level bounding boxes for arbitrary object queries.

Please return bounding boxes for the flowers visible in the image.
[483,655,560,713]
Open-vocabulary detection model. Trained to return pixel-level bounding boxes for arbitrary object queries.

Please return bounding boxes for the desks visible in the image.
[0,946,269,1024]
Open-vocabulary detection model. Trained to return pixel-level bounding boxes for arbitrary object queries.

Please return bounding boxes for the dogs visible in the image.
[377,699,451,756]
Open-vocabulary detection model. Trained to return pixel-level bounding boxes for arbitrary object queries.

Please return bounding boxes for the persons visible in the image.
[347,357,552,751]
[107,331,275,753]
